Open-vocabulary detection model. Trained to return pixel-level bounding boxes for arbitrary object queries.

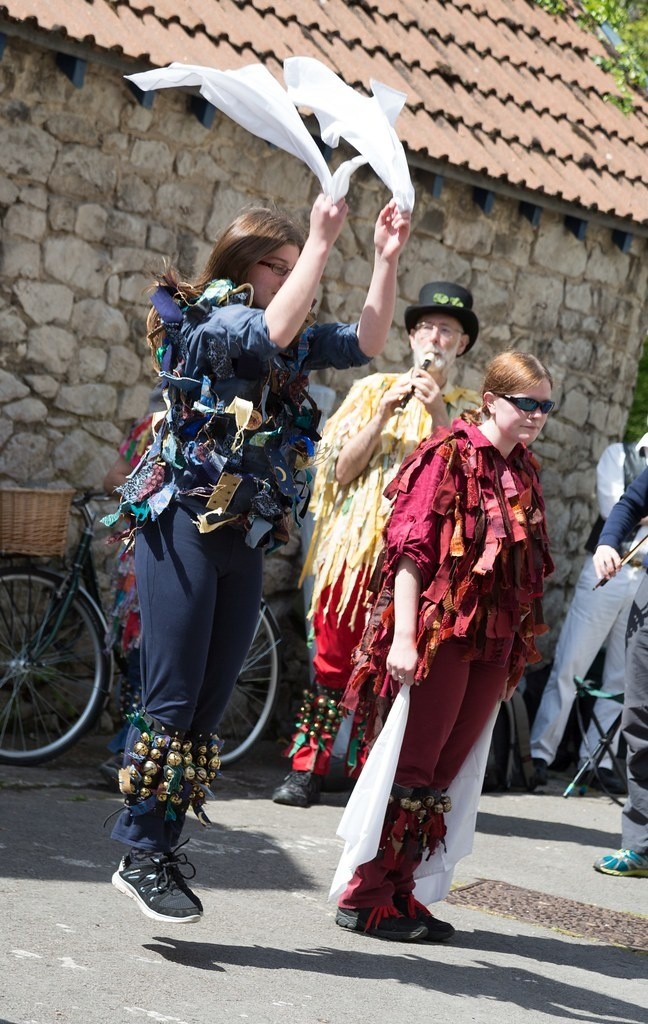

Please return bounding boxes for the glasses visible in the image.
[492,392,555,414]
[256,258,293,276]
[414,320,463,338]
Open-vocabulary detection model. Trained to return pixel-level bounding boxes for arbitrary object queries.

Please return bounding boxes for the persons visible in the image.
[594,466,648,879]
[113,191,412,924]
[102,409,169,787]
[519,440,648,796]
[334,353,553,940]
[273,281,481,807]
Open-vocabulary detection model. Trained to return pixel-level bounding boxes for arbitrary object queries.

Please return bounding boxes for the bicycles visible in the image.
[0,475,285,769]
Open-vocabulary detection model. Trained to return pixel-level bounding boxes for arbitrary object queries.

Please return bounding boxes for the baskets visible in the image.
[0,488,78,557]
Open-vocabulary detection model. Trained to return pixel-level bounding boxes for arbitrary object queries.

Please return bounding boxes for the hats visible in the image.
[405,281,479,357]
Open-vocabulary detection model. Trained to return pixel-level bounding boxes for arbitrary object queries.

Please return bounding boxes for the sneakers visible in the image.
[112,856,200,924]
[167,838,203,916]
[593,848,648,876]
[335,905,428,941]
[392,897,455,941]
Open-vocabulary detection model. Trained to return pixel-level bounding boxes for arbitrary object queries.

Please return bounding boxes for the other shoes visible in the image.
[101,750,124,793]
[526,758,547,785]
[272,770,321,806]
[575,768,628,794]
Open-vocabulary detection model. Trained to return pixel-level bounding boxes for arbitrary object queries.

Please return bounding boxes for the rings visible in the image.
[398,674,405,680]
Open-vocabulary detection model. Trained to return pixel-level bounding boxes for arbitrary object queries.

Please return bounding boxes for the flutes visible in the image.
[393,352,435,416]
